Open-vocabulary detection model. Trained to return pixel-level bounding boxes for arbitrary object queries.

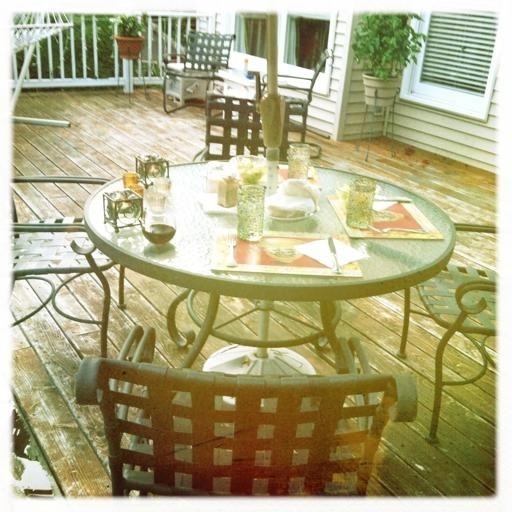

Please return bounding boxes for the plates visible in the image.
[261,203,319,221]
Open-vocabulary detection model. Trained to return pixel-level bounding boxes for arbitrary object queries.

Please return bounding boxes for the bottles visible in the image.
[243,59,248,76]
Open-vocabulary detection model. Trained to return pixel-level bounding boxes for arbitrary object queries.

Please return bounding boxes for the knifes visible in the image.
[326,235,341,274]
[369,194,413,203]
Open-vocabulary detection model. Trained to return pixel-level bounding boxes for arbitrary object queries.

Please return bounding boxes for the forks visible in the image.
[367,224,425,234]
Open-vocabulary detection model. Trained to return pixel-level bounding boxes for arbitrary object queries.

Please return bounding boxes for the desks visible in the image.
[81,156,456,408]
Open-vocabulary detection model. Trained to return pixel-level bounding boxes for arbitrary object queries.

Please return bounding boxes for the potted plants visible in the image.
[114,15,144,60]
[349,11,428,106]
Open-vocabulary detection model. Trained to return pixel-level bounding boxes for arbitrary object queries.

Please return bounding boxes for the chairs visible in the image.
[396,221,498,444]
[162,30,236,118]
[74,326,419,498]
[192,90,322,166]
[253,48,329,144]
[12,177,126,360]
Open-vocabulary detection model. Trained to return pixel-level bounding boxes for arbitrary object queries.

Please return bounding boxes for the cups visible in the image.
[122,170,144,199]
[235,184,267,243]
[143,178,171,214]
[142,203,177,245]
[288,143,309,177]
[345,176,376,230]
[237,155,267,185]
